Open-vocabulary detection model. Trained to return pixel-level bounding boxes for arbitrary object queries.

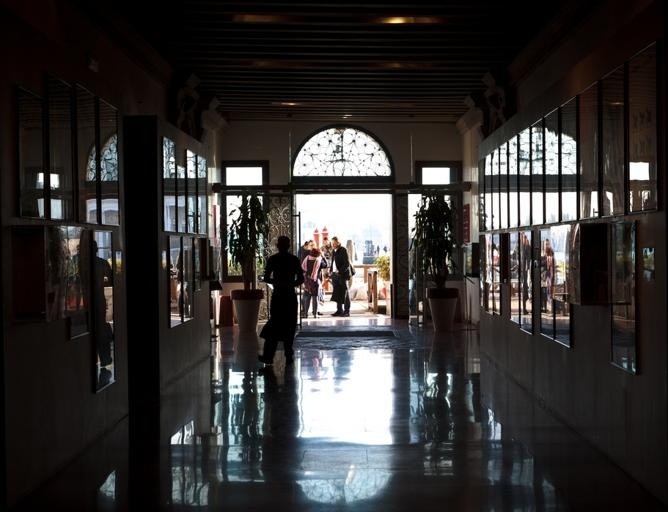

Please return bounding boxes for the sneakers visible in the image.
[258,355,274,364]
[332,310,349,316]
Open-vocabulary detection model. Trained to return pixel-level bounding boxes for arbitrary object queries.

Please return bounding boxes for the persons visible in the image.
[299,236,358,317]
[515,231,554,316]
[258,236,305,364]
[47,227,80,321]
[486,243,503,293]
[91,240,113,332]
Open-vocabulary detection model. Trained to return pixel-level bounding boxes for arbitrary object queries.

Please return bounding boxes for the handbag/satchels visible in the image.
[304,279,319,296]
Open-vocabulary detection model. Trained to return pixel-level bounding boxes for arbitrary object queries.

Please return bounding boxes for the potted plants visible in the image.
[229,192,265,333]
[409,189,461,332]
